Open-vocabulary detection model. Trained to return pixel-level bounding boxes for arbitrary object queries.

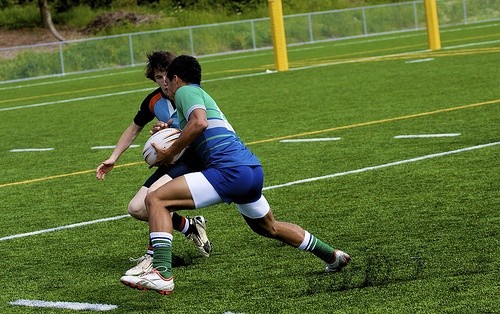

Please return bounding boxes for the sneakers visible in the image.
[121,266,175,295]
[125,254,153,276]
[185,215,212,258]
[326,249,351,271]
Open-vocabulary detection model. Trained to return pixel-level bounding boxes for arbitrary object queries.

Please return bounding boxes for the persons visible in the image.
[121,56,352,294]
[96,51,212,256]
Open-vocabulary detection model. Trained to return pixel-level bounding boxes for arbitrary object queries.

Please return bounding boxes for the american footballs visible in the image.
[141,127,188,164]
